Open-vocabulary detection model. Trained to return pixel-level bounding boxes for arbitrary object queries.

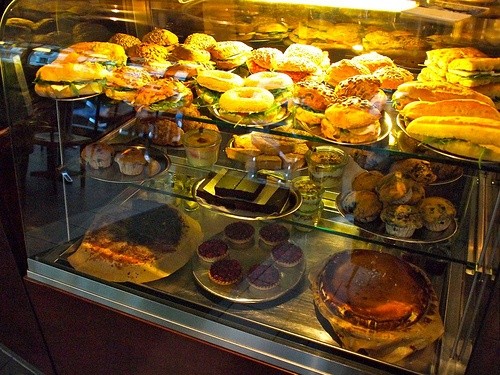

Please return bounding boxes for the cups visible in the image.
[164,179,183,207]
[183,188,198,211]
[293,200,324,232]
[304,145,349,189]
[181,128,221,167]
[290,174,325,212]
[145,180,166,203]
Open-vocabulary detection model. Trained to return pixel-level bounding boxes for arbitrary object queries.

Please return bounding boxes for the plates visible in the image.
[34,57,497,342]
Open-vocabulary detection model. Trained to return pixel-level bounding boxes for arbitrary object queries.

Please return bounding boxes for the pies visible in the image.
[317,249,430,331]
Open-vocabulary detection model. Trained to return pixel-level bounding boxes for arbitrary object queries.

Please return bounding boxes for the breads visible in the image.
[127,198,184,246]
[82,104,461,239]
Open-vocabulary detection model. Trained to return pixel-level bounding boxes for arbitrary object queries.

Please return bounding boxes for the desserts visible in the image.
[197,222,304,292]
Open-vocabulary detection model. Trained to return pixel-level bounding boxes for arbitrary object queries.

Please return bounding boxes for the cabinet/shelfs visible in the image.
[0,0,500,375]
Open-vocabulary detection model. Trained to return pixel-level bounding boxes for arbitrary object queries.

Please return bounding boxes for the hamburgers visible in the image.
[34,29,500,146]
[224,16,462,51]
